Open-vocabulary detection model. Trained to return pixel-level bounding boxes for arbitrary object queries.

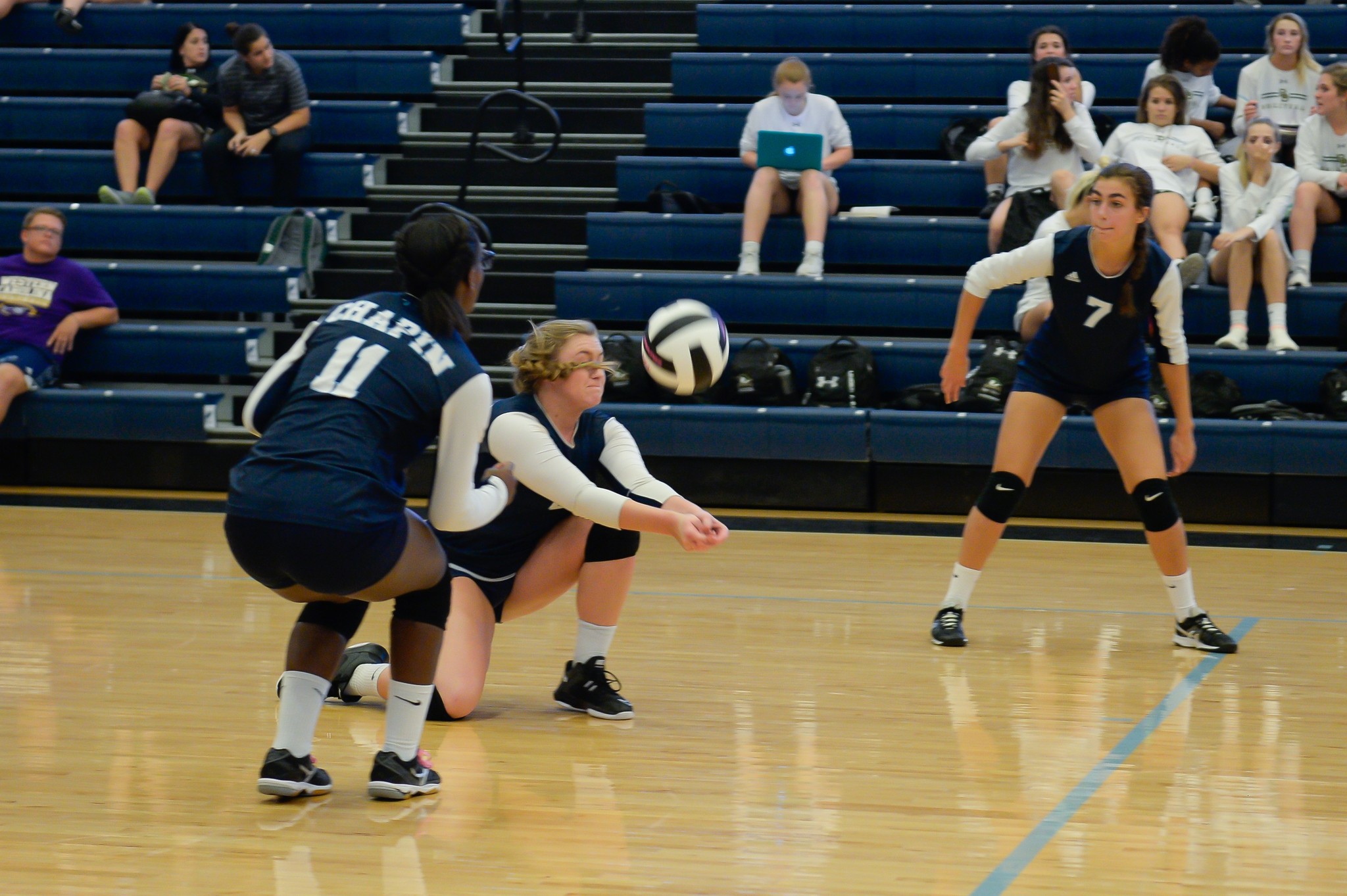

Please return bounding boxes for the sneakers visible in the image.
[1172,607,1238,653]
[366,750,441,800]
[553,655,634,721]
[277,642,390,703]
[929,605,970,647]
[255,747,334,797]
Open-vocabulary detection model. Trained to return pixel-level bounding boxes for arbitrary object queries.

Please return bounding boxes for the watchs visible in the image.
[267,126,278,137]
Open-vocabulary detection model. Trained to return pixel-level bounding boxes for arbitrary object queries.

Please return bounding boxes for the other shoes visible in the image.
[1213,331,1251,351]
[1266,330,1301,351]
[53,7,83,37]
[131,187,156,204]
[737,251,762,275]
[98,185,131,205]
[1287,264,1313,287]
[795,251,825,280]
[1177,252,1205,290]
[1187,195,1221,221]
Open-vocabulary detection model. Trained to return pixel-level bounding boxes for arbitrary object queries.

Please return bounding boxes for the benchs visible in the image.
[552,0,1347,475]
[0,0,502,443]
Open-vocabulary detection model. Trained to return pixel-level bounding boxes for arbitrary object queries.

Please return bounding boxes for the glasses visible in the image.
[27,224,63,239]
[477,248,496,270]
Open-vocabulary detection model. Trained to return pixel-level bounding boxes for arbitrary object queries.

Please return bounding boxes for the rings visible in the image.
[251,149,257,155]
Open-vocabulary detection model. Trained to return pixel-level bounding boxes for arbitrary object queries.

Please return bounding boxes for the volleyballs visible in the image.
[641,298,730,396]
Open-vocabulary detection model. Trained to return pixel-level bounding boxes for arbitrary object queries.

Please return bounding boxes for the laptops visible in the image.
[757,131,823,171]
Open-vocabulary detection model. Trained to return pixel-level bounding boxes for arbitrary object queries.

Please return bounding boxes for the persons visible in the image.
[932,161,1240,652]
[0,0,310,427]
[222,216,519,801]
[737,57,855,278]
[274,320,729,720]
[963,12,1347,351]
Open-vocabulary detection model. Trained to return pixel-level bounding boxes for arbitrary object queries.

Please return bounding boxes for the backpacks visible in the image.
[599,334,656,404]
[891,334,1347,422]
[254,206,326,299]
[995,186,1059,256]
[799,335,877,408]
[124,92,189,131]
[731,338,797,404]
[938,118,987,160]
